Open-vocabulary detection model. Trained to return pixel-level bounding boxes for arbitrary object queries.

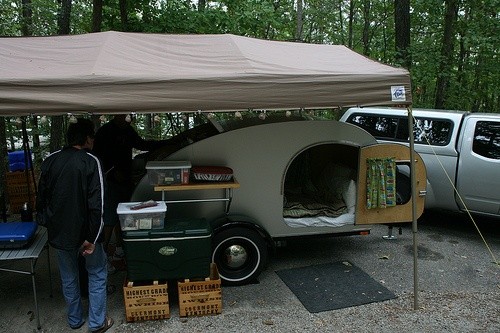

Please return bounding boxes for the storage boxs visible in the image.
[123,218,211,282]
[10,193,36,212]
[0,222,37,249]
[117,201,167,231]
[124,277,170,322]
[9,151,33,171]
[179,278,223,317]
[145,160,192,186]
[7,172,33,193]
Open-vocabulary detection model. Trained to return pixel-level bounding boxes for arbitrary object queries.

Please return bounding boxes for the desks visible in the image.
[154,181,240,216]
[0,227,52,329]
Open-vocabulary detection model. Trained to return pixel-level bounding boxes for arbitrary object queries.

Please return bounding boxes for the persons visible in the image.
[34,118,114,333]
[78,113,171,254]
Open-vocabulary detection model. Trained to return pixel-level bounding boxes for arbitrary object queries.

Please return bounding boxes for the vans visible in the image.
[337,107,500,218]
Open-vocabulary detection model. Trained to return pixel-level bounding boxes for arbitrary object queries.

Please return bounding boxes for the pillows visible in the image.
[341,179,357,210]
[310,174,343,204]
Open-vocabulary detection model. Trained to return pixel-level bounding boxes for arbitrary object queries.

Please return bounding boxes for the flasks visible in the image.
[21,200,33,222]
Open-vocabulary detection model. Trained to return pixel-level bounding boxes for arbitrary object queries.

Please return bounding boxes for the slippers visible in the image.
[92,316,113,333]
